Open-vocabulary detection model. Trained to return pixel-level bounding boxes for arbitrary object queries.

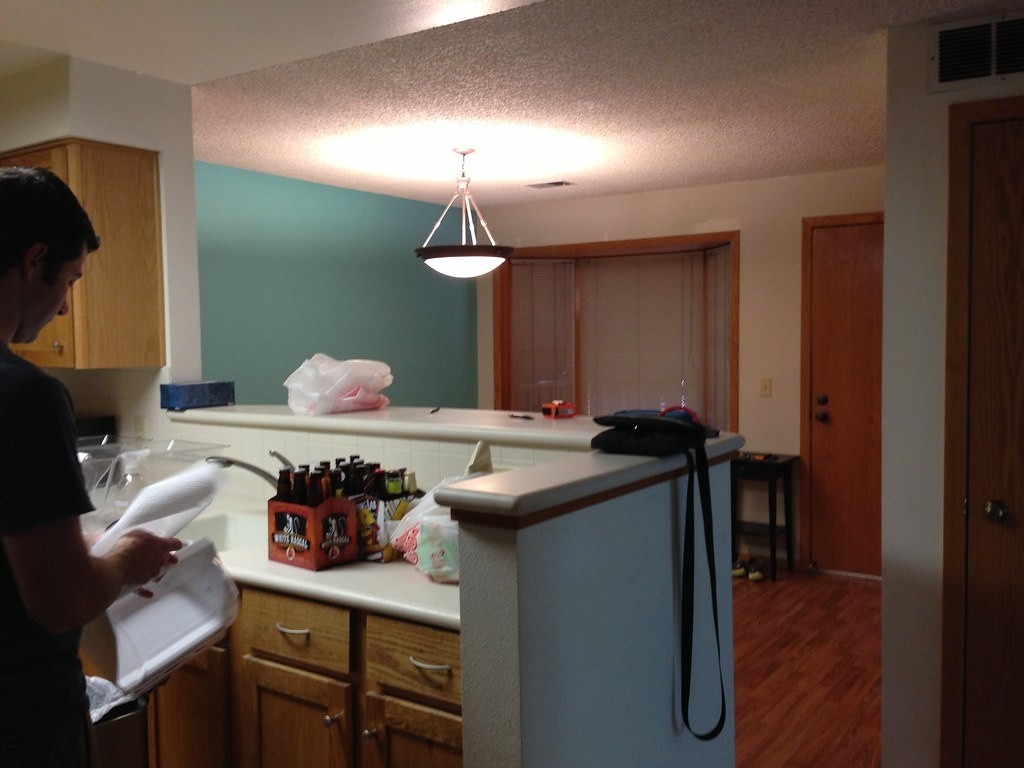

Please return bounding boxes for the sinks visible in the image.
[175,512,268,556]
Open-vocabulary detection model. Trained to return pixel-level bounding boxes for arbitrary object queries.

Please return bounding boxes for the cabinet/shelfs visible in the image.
[72,569,464,768]
[1,137,171,371]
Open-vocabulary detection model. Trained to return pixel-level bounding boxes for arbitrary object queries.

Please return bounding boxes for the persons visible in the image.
[0,166,182,768]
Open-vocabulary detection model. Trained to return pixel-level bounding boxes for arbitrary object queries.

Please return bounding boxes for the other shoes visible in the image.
[732,561,747,575]
[747,557,763,580]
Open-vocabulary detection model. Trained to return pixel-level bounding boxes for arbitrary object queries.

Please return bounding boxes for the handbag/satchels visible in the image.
[283,353,394,417]
[387,473,487,584]
[591,413,710,459]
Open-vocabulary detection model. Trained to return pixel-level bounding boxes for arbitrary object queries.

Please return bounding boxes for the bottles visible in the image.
[272,453,418,548]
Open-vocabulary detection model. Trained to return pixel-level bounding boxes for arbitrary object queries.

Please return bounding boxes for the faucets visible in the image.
[205,449,295,490]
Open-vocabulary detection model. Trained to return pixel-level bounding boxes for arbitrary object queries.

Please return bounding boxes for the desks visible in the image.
[735,449,799,581]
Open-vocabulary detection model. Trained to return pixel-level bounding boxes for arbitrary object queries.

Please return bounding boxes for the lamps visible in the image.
[416,149,511,279]
